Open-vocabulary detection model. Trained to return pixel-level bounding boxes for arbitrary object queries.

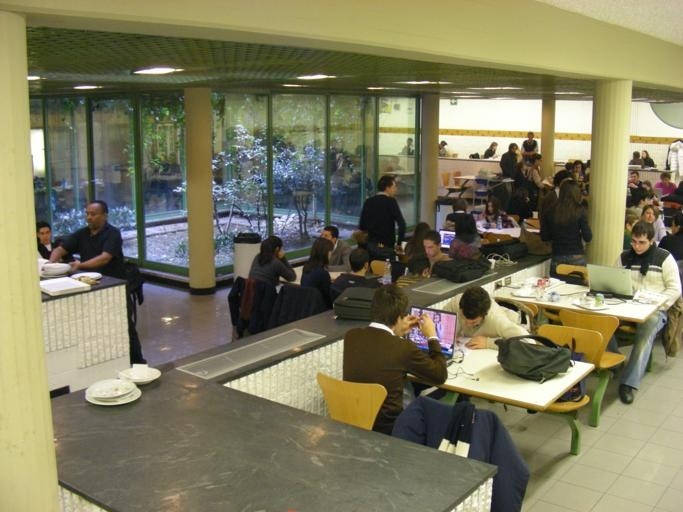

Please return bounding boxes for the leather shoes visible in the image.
[618,385,634,405]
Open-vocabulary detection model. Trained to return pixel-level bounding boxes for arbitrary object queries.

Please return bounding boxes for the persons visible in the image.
[555,161,590,192]
[439,141,448,157]
[626,172,683,261]
[249,236,296,287]
[450,213,481,258]
[481,196,514,229]
[301,237,334,297]
[48,201,146,365]
[444,286,536,351]
[607,221,682,404]
[629,150,654,168]
[359,175,406,262]
[37,222,74,263]
[540,180,592,275]
[397,223,449,288]
[446,199,467,232]
[343,283,449,434]
[322,225,352,273]
[402,138,414,155]
[330,248,379,300]
[485,142,498,159]
[499,132,545,223]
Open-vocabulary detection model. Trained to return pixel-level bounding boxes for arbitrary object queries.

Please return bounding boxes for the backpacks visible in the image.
[495,334,571,383]
[481,239,530,260]
[434,255,491,282]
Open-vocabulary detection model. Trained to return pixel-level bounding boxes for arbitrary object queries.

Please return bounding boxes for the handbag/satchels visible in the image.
[334,286,385,320]
[553,272,585,285]
[556,352,586,403]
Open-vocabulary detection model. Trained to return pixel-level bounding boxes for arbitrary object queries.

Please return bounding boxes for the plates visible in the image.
[70,271,102,280]
[510,288,539,298]
[547,284,584,296]
[84,378,142,407]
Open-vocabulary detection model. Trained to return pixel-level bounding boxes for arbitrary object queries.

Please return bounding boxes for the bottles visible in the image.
[651,192,656,201]
[535,279,545,301]
[579,291,594,307]
[496,216,502,231]
[382,258,392,286]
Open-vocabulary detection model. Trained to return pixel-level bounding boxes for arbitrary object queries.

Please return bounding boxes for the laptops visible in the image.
[439,229,456,249]
[407,306,456,360]
[587,264,634,299]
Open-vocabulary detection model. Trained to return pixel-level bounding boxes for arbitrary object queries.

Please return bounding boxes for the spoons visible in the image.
[111,368,139,381]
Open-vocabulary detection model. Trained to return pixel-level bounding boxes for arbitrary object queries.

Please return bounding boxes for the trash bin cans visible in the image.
[233,233,262,285]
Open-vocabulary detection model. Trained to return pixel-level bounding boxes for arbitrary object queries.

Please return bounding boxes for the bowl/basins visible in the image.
[119,366,161,385]
[43,263,71,274]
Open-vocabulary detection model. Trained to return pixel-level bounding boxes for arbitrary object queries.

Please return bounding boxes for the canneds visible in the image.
[594,293,605,305]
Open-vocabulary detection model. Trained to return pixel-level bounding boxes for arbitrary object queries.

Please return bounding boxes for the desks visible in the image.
[279,169,669,288]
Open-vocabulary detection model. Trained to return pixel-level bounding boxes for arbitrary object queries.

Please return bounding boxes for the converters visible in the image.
[488,259,496,269]
[587,291,614,298]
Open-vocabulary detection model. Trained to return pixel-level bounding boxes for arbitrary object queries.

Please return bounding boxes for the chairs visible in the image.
[318,261,669,456]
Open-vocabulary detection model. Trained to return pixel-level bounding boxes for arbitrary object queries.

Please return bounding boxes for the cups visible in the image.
[453,171,462,188]
[441,171,451,187]
[487,259,495,269]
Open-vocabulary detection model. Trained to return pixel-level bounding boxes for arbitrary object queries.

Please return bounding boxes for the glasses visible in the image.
[629,239,649,246]
